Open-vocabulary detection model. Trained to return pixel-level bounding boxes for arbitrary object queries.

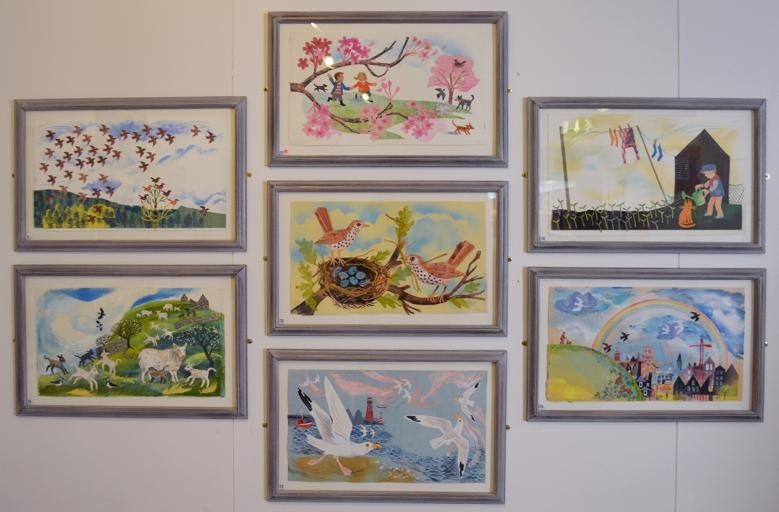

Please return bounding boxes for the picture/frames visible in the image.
[265,9,509,169]
[12,264,249,421]
[265,179,508,337]
[527,266,767,423]
[266,347,508,506]
[12,95,248,253]
[526,96,767,255]
